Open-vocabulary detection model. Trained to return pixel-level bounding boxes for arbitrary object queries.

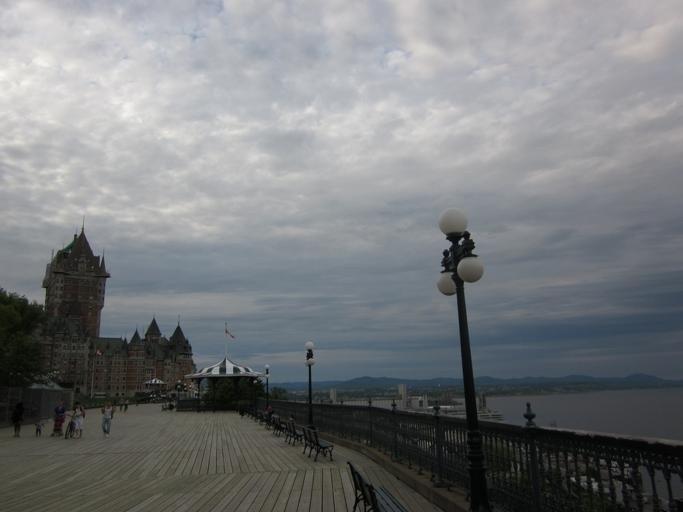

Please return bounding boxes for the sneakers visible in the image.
[104,432,110,438]
[74,436,82,440]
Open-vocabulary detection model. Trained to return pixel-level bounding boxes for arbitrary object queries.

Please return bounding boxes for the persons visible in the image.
[71,401,83,440]
[118,397,123,412]
[34,420,44,437]
[123,398,129,412]
[50,399,64,438]
[10,401,24,437]
[100,398,114,439]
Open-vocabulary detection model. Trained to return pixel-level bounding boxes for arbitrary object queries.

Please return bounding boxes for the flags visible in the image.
[226,329,238,342]
[95,348,103,358]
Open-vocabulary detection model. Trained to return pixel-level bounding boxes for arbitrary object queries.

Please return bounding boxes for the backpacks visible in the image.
[111,410,114,419]
[83,414,85,418]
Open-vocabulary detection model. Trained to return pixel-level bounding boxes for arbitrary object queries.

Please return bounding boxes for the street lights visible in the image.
[303,340,316,425]
[263,364,270,413]
[438,210,492,511]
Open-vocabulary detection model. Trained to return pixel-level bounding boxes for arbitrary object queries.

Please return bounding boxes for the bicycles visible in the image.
[64,412,76,438]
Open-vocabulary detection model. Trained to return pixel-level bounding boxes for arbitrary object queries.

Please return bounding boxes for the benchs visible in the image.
[347,461,409,512]
[244,406,304,446]
[302,426,334,462]
